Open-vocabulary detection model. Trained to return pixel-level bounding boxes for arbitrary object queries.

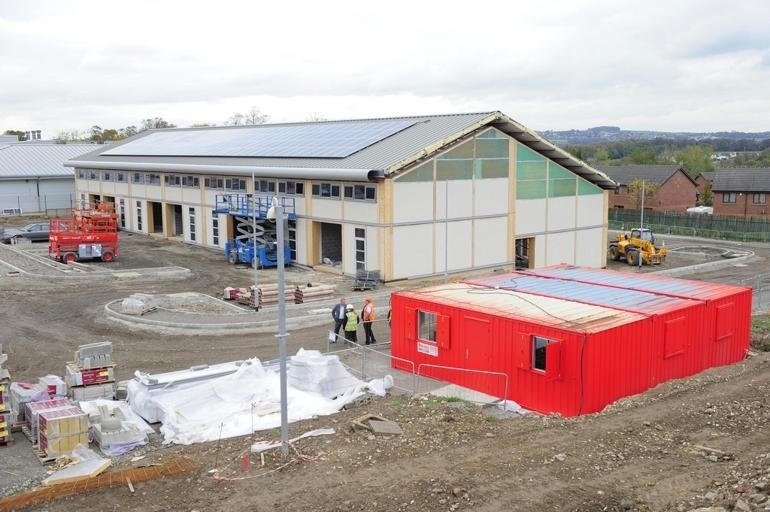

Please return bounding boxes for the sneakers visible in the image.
[332,338,377,349]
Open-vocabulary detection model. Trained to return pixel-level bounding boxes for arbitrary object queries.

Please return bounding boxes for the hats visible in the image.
[365,295,372,303]
[346,304,354,309]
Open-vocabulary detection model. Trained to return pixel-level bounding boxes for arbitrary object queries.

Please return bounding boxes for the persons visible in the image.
[329,298,348,345]
[360,297,377,346]
[343,303,361,345]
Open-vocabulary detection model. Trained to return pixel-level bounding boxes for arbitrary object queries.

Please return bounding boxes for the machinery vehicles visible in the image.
[609,228,667,266]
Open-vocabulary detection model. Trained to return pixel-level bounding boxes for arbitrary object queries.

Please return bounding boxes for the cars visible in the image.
[2,223,65,244]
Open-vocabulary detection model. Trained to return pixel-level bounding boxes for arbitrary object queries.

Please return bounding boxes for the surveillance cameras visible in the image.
[267,206,278,223]
